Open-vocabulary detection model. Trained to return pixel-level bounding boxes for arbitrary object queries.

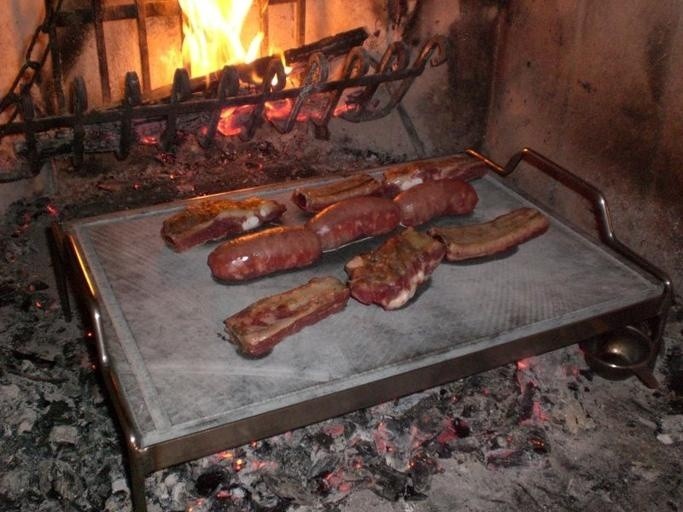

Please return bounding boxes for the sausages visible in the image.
[306,194,399,247]
[208,225,320,281]
[393,177,478,226]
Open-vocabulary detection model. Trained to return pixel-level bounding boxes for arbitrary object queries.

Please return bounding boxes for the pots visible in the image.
[580,324,661,390]
